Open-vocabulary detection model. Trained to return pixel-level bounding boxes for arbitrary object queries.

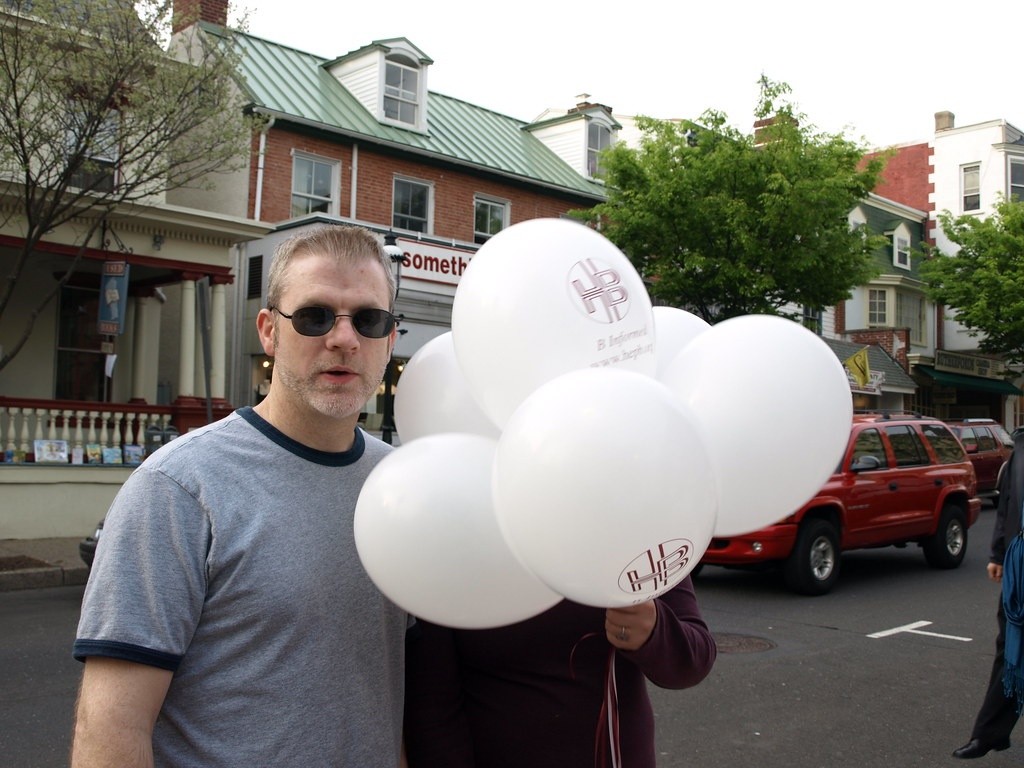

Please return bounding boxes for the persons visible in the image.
[400,564,715,768]
[70,223,415,768]
[953,434,1024,758]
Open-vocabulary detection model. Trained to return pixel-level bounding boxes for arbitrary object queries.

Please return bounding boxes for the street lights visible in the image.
[382,228,407,445]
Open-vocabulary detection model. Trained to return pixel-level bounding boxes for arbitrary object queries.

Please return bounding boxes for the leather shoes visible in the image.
[951,735,1012,759]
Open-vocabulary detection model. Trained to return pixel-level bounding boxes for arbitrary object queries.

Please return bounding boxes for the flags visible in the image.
[846,347,871,389]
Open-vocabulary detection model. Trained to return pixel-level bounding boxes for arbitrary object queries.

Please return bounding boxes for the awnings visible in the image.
[919,363,1024,395]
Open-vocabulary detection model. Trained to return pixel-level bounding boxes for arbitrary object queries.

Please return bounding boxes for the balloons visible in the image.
[643,304,715,391]
[492,370,717,614]
[354,433,564,629]
[682,314,853,538]
[452,217,654,429]
[395,331,503,441]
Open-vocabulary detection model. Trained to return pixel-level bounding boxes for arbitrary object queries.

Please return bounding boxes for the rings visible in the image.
[620,625,627,640]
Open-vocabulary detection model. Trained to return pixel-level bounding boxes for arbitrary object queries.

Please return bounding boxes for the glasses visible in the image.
[270,306,400,339]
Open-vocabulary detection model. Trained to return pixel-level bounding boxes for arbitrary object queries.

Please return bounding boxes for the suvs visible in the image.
[690,410,982,597]
[942,417,1015,510]
